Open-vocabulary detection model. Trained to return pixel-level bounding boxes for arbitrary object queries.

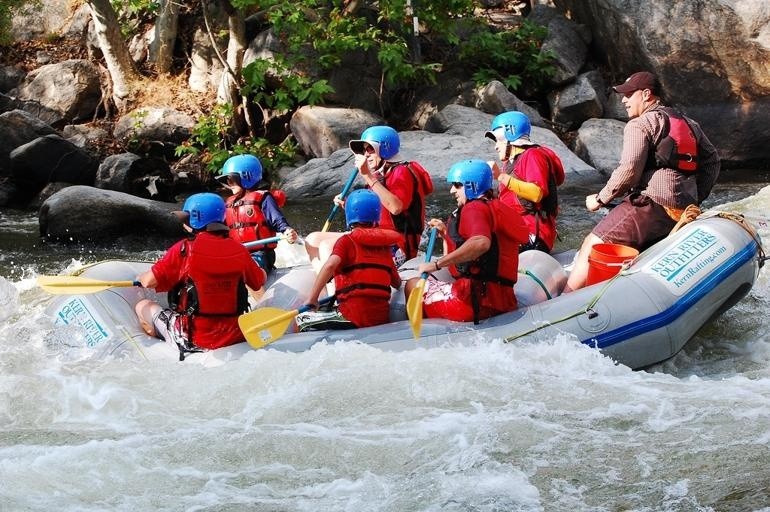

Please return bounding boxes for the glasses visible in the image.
[452,180,463,190]
[358,144,375,156]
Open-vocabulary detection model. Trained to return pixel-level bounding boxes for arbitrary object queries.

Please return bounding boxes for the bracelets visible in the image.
[371,180,378,188]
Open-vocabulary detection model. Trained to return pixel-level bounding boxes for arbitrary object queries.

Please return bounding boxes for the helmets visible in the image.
[182,193,226,230]
[491,111,531,142]
[223,154,263,189]
[446,159,493,200]
[344,188,381,230]
[360,125,401,160]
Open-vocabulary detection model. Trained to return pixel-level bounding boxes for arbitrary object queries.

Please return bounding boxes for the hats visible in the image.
[170,211,231,233]
[613,71,661,95]
[215,173,269,190]
[349,139,405,163]
[484,128,537,148]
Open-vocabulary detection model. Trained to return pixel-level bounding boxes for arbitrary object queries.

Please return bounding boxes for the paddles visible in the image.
[37,276,142,295]
[406,220,438,339]
[238,293,336,349]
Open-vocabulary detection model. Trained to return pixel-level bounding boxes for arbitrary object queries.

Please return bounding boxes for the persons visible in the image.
[559,71,722,296]
[305,125,434,301]
[484,111,565,254]
[292,188,404,333]
[404,159,530,322]
[214,154,298,274]
[135,192,267,352]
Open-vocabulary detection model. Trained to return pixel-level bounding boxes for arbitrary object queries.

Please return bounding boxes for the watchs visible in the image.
[434,259,441,270]
[595,195,604,206]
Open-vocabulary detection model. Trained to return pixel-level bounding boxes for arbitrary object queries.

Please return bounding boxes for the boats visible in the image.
[50,212,770,370]
